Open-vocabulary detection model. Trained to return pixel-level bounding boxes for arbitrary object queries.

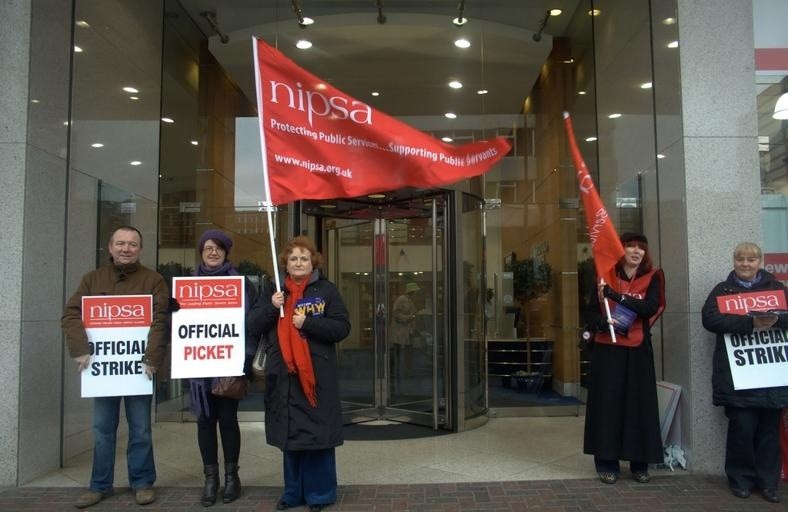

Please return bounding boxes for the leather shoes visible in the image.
[731,486,750,497]
[277,500,289,510]
[761,489,779,502]
[309,504,323,512]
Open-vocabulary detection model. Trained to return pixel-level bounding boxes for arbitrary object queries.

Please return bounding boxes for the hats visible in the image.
[620,231,647,243]
[405,283,420,294]
[198,229,232,252]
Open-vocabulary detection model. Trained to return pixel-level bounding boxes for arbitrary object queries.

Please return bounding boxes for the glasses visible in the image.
[202,245,223,253]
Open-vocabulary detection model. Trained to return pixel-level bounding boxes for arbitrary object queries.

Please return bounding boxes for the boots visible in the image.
[222,463,241,503]
[201,463,220,507]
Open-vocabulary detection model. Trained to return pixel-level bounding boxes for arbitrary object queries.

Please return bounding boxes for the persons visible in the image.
[700,241,788,503]
[580,232,666,485]
[60,225,168,509]
[248,237,351,512]
[168,231,257,508]
[389,283,422,375]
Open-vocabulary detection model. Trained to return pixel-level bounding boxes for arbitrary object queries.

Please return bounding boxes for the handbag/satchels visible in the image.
[210,374,248,401]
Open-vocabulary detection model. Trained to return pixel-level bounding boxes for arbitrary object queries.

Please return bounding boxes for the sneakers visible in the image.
[634,471,651,483]
[74,490,103,507]
[135,487,154,503]
[597,470,616,484]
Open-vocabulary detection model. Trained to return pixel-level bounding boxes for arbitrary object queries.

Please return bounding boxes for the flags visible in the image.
[565,118,627,285]
[251,36,512,208]
